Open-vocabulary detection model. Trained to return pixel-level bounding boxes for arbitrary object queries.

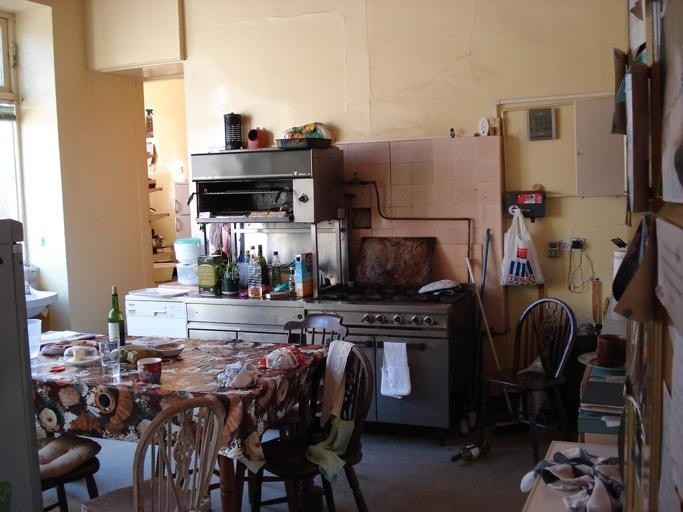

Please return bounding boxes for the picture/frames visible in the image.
[624,278,662,510]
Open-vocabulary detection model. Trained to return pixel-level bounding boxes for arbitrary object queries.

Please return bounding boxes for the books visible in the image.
[576,380,625,418]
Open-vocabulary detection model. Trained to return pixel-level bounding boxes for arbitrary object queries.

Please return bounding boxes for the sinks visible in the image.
[24,282,58,319]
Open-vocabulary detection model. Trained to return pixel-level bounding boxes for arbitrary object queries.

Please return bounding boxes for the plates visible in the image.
[65,356,98,366]
[576,351,625,372]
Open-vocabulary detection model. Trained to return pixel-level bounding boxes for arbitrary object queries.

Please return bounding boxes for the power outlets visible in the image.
[569,238,584,252]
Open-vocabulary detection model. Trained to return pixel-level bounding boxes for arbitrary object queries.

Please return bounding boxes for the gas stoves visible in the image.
[304,283,474,326]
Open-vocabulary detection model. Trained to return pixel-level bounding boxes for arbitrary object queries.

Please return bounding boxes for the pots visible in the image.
[174,238,200,263]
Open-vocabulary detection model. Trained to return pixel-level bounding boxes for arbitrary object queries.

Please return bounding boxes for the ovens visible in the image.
[188,149,344,225]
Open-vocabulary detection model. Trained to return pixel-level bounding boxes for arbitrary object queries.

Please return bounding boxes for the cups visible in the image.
[98,338,119,367]
[136,357,162,381]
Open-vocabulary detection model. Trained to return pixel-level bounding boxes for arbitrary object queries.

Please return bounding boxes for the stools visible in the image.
[35,434,101,511]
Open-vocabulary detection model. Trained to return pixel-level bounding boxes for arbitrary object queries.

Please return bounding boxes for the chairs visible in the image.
[281,314,349,436]
[474,297,575,468]
[80,398,224,512]
[233,341,376,510]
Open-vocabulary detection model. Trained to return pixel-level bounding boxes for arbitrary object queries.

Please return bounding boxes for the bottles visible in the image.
[287,267,295,296]
[151,235,157,254]
[448,440,489,462]
[236,245,281,297]
[458,384,477,437]
[106,285,125,347]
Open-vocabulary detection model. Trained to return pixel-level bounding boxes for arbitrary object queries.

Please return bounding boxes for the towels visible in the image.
[318,339,357,426]
[380,341,412,400]
[305,417,355,484]
[216,360,261,388]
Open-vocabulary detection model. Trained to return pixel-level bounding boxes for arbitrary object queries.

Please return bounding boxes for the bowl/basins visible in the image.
[154,343,184,357]
[151,263,175,283]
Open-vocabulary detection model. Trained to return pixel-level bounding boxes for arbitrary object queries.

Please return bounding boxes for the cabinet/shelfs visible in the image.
[145,132,170,224]
[186,304,305,342]
[520,440,625,511]
[125,287,199,339]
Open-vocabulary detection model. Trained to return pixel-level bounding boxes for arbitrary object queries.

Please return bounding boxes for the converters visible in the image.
[573,241,581,250]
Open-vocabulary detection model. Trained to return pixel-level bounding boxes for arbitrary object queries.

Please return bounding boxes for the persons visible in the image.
[151,227,165,255]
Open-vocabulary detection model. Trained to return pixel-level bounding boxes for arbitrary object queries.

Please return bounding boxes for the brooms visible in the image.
[465,258,521,440]
